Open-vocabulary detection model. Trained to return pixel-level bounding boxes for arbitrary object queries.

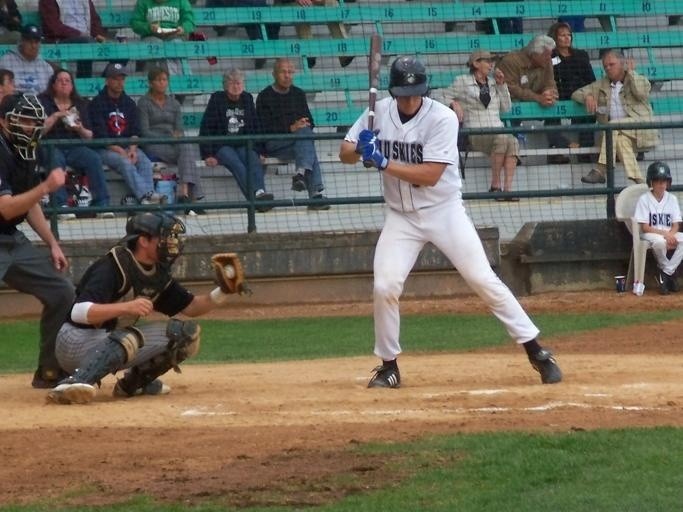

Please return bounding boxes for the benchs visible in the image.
[0,0,683,242]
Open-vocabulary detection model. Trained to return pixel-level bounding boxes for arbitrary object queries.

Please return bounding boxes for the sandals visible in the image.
[489,185,520,202]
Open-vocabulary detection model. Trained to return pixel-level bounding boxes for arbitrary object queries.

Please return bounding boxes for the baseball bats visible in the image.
[363,35,381,168]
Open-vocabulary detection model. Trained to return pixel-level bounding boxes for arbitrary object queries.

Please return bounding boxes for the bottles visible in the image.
[517,122,526,146]
[152,163,160,179]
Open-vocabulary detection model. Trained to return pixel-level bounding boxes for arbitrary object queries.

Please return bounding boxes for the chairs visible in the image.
[614,184,683,292]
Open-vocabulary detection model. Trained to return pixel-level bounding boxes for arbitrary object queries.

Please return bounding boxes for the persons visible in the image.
[0,69,16,104]
[37,69,115,220]
[47,213,236,404]
[0,25,54,96]
[138,67,206,215]
[634,162,683,294]
[199,69,274,212]
[340,55,562,388]
[0,92,74,388]
[92,63,168,205]
[256,60,331,210]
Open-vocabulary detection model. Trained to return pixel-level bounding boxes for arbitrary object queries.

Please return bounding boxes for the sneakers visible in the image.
[306,195,331,211]
[579,168,607,184]
[367,365,401,390]
[44,383,97,407]
[111,376,171,400]
[58,196,202,221]
[528,348,562,384]
[292,173,306,192]
[654,271,669,295]
[30,365,69,388]
[254,191,274,211]
[546,146,598,164]
[669,274,680,292]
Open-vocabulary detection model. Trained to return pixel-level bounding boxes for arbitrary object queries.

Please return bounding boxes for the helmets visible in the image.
[0,90,49,163]
[388,55,429,96]
[117,207,187,272]
[645,161,673,189]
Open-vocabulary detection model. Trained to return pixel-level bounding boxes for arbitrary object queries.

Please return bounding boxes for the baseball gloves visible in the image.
[210,253,250,294]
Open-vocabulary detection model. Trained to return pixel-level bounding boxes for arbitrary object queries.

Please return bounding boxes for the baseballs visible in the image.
[224,265,236,279]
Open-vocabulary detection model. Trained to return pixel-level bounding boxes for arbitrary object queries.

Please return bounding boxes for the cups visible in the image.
[614,275,627,292]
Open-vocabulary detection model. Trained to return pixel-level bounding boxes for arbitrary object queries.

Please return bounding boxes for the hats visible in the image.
[21,24,43,40]
[468,50,499,67]
[100,61,129,79]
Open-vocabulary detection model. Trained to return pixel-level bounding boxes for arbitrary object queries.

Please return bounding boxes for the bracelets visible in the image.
[210,286,228,304]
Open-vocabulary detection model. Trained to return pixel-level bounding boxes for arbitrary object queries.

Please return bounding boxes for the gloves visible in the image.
[362,139,389,171]
[355,128,381,156]
